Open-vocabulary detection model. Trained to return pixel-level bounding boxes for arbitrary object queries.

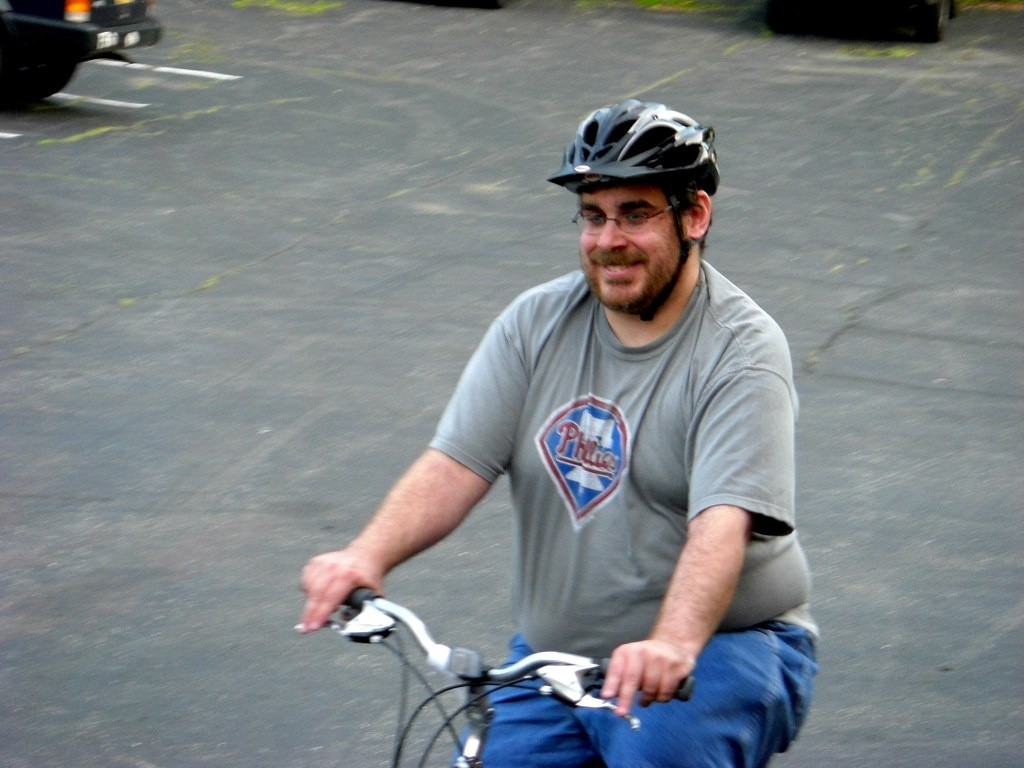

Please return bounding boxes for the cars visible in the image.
[0,0,163,109]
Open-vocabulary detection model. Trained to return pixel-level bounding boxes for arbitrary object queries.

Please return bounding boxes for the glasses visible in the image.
[572,200,680,235]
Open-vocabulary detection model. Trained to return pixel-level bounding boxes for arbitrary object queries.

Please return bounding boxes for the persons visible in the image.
[295,96,818,768]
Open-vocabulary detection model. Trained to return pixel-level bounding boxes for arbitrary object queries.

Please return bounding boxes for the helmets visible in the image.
[546,99,720,197]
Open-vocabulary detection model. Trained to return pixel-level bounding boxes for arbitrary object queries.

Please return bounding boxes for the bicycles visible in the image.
[293,585,695,768]
